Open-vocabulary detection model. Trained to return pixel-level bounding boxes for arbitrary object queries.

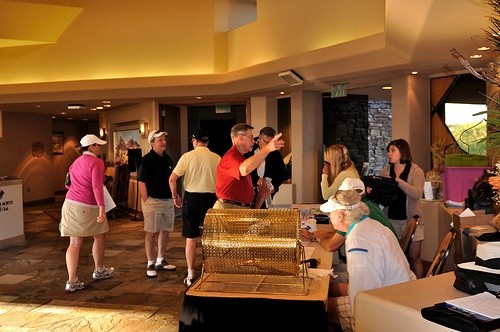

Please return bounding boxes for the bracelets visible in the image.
[311,235,315,242]
[171,195,179,199]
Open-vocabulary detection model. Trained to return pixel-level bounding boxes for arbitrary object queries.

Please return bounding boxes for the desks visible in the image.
[353,272,500,332]
[180,202,331,332]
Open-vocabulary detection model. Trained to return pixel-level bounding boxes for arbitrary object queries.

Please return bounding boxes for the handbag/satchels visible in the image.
[65,172,71,186]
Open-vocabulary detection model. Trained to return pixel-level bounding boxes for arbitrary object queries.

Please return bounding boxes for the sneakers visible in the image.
[65,277,85,292]
[146,263,157,276]
[92,266,115,278]
[155,260,177,270]
[184,273,195,287]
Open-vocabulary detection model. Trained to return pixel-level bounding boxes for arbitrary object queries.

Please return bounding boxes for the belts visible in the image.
[218,198,254,207]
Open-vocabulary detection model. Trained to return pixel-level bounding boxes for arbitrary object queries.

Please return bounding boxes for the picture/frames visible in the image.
[111,129,140,171]
[52,131,64,155]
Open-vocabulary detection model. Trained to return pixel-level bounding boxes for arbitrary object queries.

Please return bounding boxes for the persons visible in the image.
[365,139,425,277]
[138,129,178,278]
[320,189,417,296]
[295,175,398,296]
[211,122,285,211]
[243,127,290,210]
[166,128,223,289]
[57,133,115,291]
[320,142,362,201]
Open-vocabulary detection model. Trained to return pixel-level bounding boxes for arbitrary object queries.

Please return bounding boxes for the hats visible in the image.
[191,126,211,142]
[319,195,362,213]
[80,134,108,147]
[338,177,367,196]
[147,130,169,143]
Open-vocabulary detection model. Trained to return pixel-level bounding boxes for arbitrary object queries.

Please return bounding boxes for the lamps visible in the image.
[139,122,149,139]
[100,128,106,140]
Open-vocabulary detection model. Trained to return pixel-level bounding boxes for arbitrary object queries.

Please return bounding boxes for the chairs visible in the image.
[399,215,420,254]
[424,249,449,277]
[432,228,457,272]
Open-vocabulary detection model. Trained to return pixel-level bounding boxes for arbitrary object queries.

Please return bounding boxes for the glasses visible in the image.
[260,135,270,144]
[239,134,254,140]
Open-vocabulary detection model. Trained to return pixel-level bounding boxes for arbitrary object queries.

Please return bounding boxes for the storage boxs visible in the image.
[454,209,496,263]
[442,166,495,207]
